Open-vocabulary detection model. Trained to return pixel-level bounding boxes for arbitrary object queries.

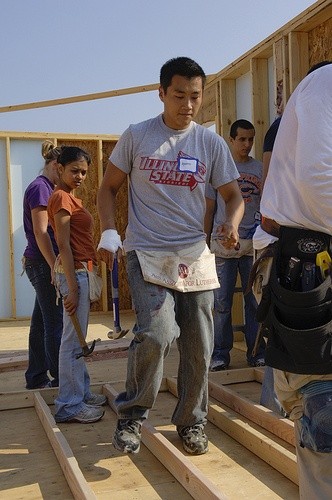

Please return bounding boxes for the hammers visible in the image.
[64,296,96,359]
[107,251,129,340]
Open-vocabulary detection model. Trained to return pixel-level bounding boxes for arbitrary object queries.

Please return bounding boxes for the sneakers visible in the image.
[177,423,208,454]
[210,360,230,371]
[54,403,105,422]
[113,418,141,455]
[25,379,59,388]
[85,394,107,405]
[250,359,266,367]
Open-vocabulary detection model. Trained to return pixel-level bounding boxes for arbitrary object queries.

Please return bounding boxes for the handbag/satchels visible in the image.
[294,442,332,500]
[81,261,103,301]
[134,241,221,292]
[246,226,332,375]
[210,234,255,258]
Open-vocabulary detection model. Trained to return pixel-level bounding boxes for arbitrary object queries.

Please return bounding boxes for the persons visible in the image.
[256,58,332,500]
[48,146,108,423]
[94,57,243,457]
[205,119,261,370]
[20,140,62,388]
